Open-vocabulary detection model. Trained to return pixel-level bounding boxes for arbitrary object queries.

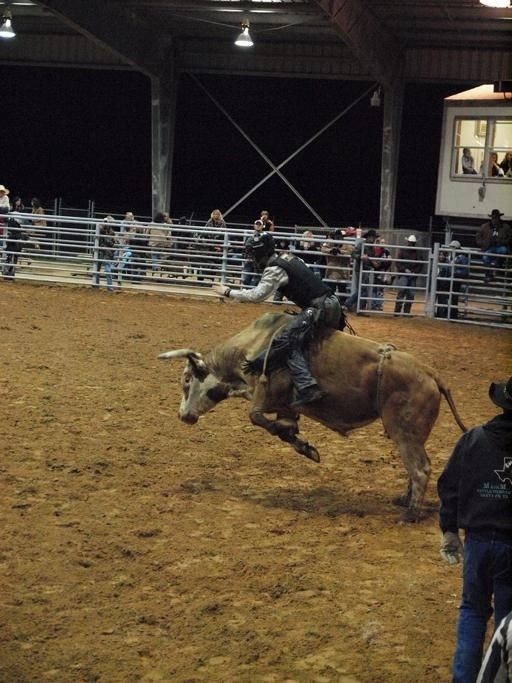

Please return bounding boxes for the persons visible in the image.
[90,210,274,294]
[1,185,48,281]
[479,152,504,177]
[498,151,512,175]
[462,148,477,175]
[211,231,347,403]
[275,209,512,323]
[435,377,512,683]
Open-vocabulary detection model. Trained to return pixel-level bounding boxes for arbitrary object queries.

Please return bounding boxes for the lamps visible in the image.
[234,14,254,48]
[0,0,16,39]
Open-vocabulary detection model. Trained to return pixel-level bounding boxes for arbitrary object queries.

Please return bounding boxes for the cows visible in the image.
[156,311,469,525]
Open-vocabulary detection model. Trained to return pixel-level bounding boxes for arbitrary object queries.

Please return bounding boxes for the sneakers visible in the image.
[289,384,323,409]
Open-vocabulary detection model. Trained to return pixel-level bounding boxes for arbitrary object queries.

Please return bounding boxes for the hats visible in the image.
[488,209,505,217]
[330,225,380,240]
[103,215,115,222]
[447,239,462,249]
[0,185,10,196]
[404,233,421,244]
[488,376,512,409]
[242,233,283,256]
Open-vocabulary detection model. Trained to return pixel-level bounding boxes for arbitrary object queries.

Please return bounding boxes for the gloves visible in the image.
[439,531,465,564]
